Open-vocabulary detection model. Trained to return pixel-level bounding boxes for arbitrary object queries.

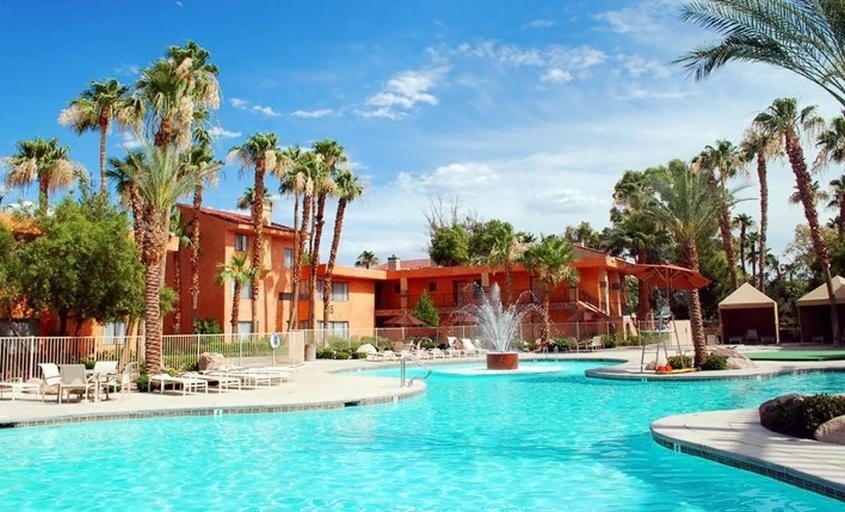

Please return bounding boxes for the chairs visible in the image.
[366,336,490,361]
[639,305,684,372]
[580,336,603,351]
[729,329,774,344]
[0,360,296,406]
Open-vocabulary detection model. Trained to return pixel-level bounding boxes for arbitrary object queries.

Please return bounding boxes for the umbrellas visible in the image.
[618,257,713,319]
